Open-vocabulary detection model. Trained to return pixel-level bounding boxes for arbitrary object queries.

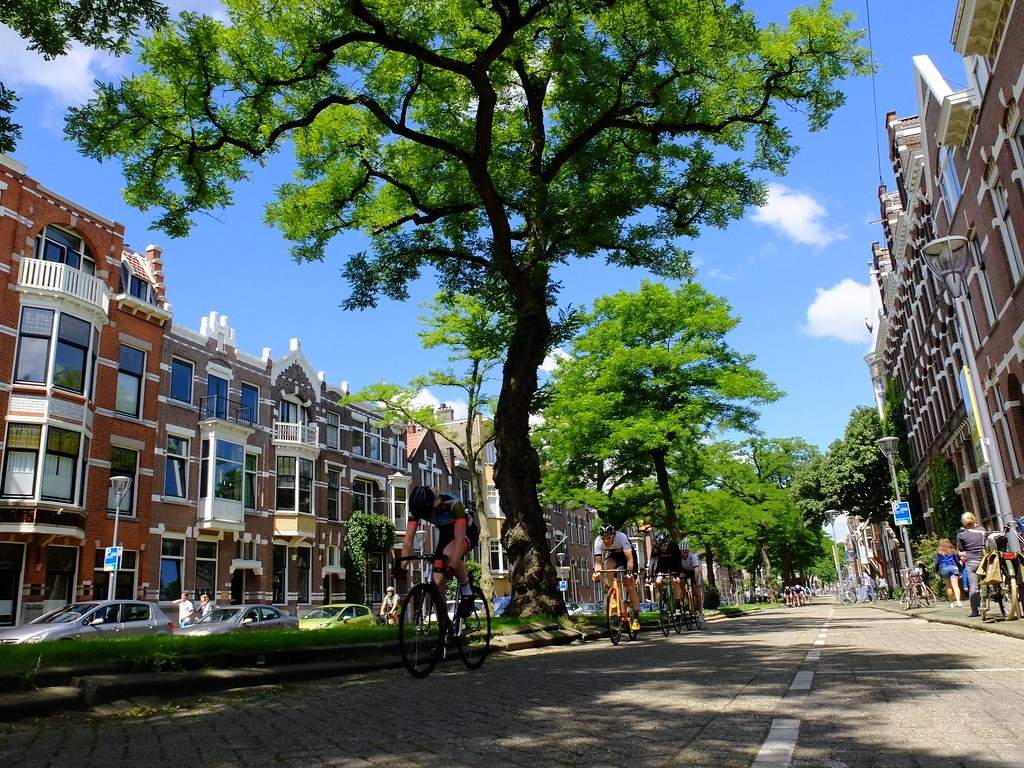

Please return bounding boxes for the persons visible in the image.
[645,532,706,625]
[783,584,812,606]
[860,567,890,604]
[399,485,480,620]
[377,586,401,626]
[171,590,215,627]
[591,523,642,631]
[934,510,991,617]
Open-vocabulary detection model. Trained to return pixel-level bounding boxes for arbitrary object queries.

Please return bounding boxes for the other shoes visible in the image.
[699,612,705,623]
[661,599,669,611]
[675,608,682,617]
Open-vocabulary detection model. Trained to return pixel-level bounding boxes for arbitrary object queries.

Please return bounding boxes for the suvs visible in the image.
[0,599,175,645]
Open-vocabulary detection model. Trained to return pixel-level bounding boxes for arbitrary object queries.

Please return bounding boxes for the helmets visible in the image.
[599,522,616,535]
[409,485,435,519]
[655,531,671,544]
[387,586,394,591]
[678,542,690,551]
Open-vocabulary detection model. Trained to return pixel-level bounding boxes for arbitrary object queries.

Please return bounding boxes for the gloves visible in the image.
[393,568,406,579]
[443,565,455,580]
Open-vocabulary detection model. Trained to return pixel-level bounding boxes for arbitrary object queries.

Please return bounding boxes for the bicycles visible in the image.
[596,567,637,645]
[375,612,394,626]
[390,554,492,678]
[977,515,1024,622]
[648,571,682,636]
[842,587,888,606]
[786,595,811,608]
[899,562,937,610]
[674,573,702,631]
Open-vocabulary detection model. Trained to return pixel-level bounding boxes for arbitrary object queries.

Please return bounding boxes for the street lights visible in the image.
[825,510,843,592]
[877,436,921,609]
[723,578,727,597]
[921,233,1023,560]
[556,553,566,600]
[109,476,133,599]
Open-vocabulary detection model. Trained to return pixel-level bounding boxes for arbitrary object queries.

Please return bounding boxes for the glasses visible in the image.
[680,551,687,554]
[601,534,613,540]
[658,543,667,547]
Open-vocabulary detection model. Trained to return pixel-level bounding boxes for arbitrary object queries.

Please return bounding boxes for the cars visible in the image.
[600,600,659,612]
[423,596,511,624]
[173,605,299,637]
[744,594,767,603]
[579,604,605,616]
[565,602,582,616]
[719,598,735,606]
[296,603,376,630]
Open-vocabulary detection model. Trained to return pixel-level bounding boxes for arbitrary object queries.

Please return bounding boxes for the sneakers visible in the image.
[631,619,641,631]
[459,590,476,618]
[611,596,618,609]
[429,639,453,657]
